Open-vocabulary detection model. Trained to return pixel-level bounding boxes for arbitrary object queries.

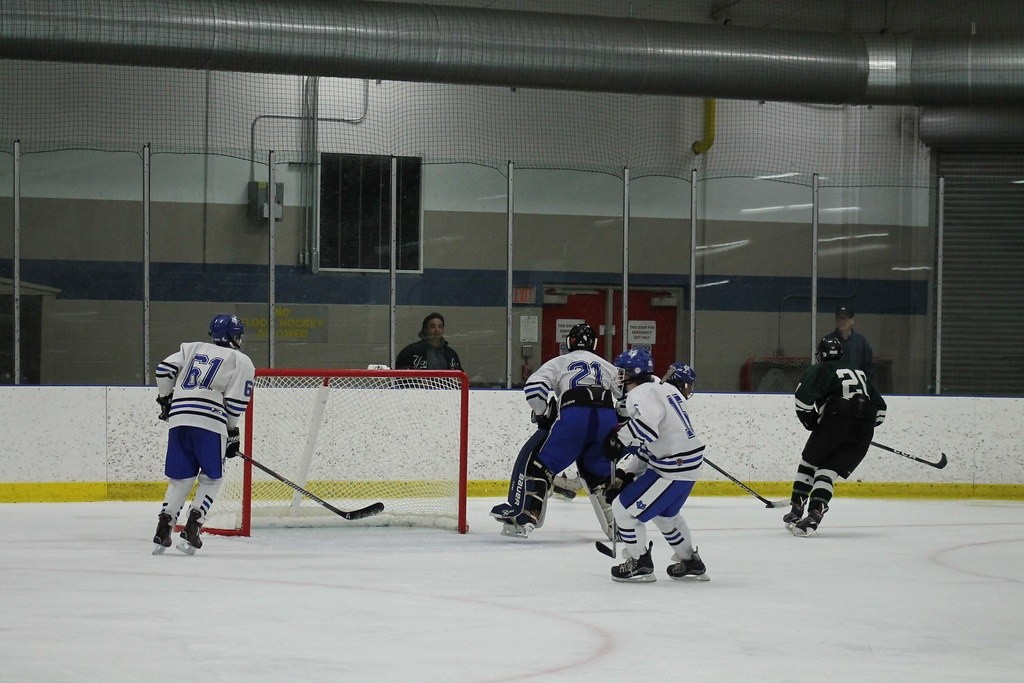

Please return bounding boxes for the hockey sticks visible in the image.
[869,439,948,471]
[700,454,791,509]
[595,460,618,558]
[233,449,385,520]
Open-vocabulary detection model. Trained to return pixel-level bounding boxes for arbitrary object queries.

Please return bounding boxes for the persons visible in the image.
[151,314,255,556]
[606,346,710,583]
[548,362,699,504]
[487,322,643,541]
[782,337,887,537]
[816,305,873,384]
[395,312,463,390]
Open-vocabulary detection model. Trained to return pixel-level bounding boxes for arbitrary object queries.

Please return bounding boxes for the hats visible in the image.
[836,306,853,319]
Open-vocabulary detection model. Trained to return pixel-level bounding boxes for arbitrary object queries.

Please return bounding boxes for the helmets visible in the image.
[615,348,653,383]
[566,323,597,351]
[815,336,846,363]
[209,313,245,348]
[667,362,695,398]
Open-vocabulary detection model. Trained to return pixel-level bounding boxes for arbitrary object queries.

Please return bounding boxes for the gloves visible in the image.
[602,469,635,504]
[802,412,820,430]
[603,426,626,462]
[156,391,173,420]
[225,427,240,458]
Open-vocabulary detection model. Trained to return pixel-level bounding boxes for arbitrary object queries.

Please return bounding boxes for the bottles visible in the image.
[368,365,391,370]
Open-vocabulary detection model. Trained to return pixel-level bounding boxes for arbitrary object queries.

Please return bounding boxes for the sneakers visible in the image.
[176,507,204,555]
[152,513,173,555]
[611,540,657,583]
[783,496,808,530]
[667,545,710,581]
[500,504,539,537]
[793,503,829,537]
